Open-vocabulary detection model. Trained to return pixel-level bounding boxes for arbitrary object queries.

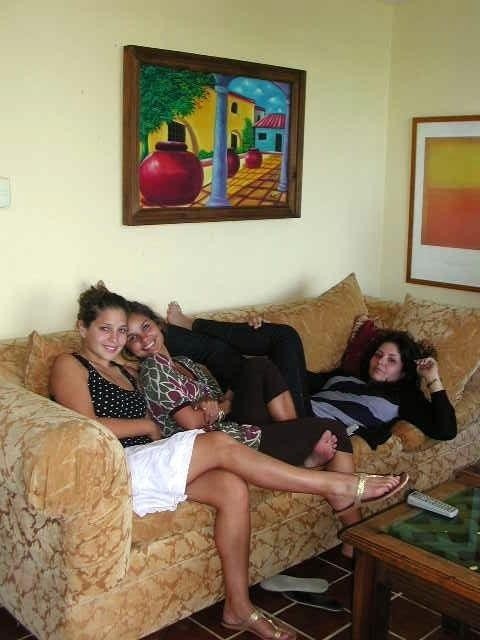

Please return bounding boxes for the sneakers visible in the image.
[259,574,345,612]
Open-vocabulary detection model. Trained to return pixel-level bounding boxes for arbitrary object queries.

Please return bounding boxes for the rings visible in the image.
[202,407,208,410]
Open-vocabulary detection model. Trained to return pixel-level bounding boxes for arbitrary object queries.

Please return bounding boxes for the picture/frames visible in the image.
[407,117,480,292]
[123,44,307,226]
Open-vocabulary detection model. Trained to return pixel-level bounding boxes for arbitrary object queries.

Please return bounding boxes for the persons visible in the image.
[115,300,364,560]
[95,277,458,451]
[48,284,411,640]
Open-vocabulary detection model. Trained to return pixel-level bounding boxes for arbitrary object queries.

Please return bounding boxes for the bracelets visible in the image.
[426,377,440,389]
[216,407,225,424]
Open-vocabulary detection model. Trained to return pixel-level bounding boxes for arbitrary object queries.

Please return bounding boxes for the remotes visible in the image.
[406,488,459,520]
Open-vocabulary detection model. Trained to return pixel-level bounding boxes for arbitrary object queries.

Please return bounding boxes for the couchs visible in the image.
[1,297,480,639]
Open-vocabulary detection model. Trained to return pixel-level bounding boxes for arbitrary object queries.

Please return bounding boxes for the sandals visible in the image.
[332,471,410,516]
[220,606,297,640]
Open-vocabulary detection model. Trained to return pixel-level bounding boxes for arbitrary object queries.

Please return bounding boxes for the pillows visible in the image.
[26,331,74,400]
[258,272,480,419]
